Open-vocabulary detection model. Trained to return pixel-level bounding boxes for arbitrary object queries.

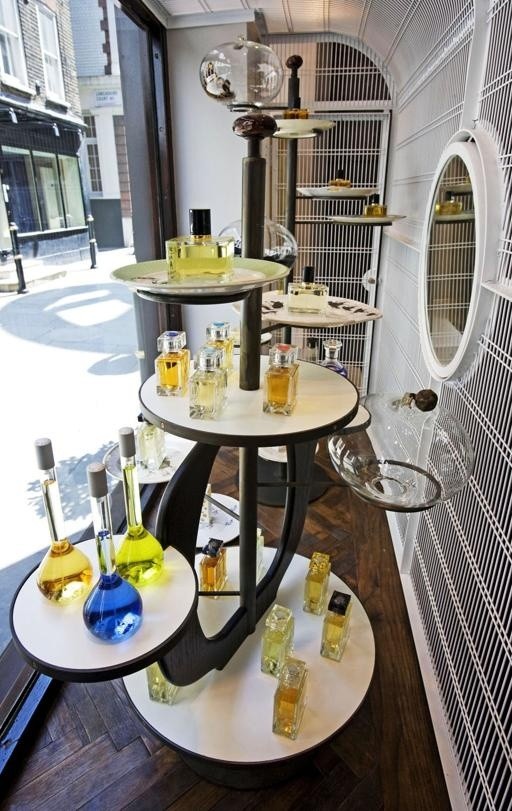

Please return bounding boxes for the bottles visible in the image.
[315,339,348,378]
[262,340,296,418]
[434,191,462,218]
[136,414,166,469]
[147,663,179,707]
[284,99,309,120]
[259,604,316,740]
[366,191,387,217]
[194,537,228,596]
[330,168,348,186]
[303,553,356,663]
[286,264,328,317]
[30,428,163,647]
[164,208,234,287]
[157,321,234,424]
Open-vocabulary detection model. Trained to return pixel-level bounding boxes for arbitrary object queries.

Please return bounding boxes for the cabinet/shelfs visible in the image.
[7,53,475,789]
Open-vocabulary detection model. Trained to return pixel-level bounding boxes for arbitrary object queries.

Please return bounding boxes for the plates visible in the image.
[110,254,288,296]
[230,288,384,333]
[328,211,404,227]
[291,183,387,198]
[225,334,272,350]
[266,118,341,141]
[99,437,186,487]
